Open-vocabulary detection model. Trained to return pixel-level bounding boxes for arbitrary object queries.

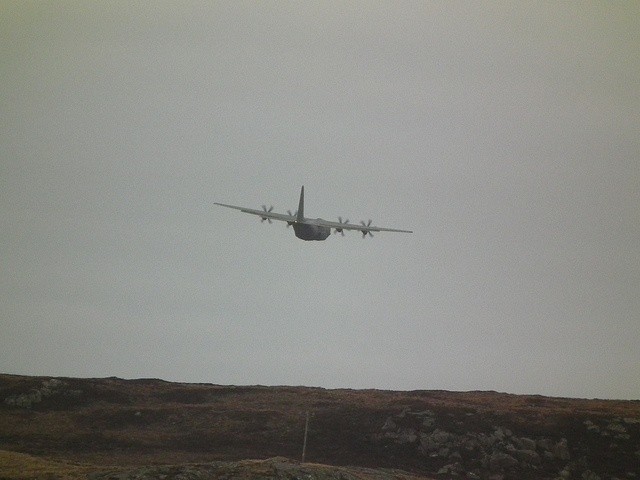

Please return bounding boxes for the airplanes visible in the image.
[213,185,413,241]
